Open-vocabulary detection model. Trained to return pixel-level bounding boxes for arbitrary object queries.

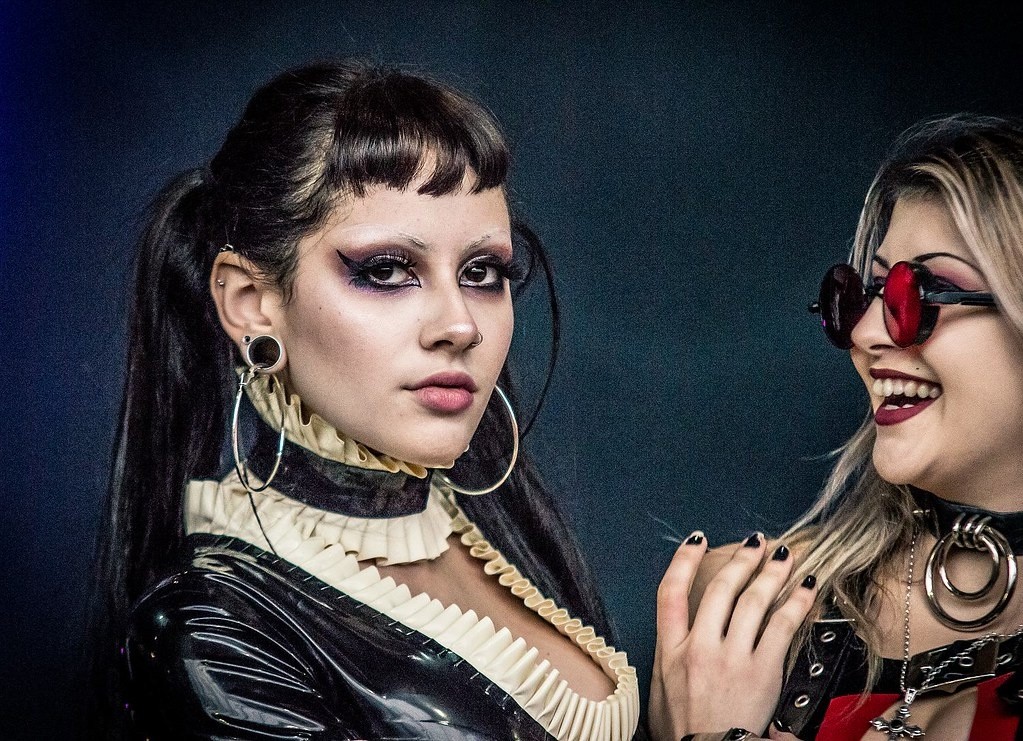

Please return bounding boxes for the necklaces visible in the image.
[862,522,1019,741]
[912,483,1023,633]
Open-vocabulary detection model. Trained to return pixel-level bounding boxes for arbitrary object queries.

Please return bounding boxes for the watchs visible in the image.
[681,727,764,741]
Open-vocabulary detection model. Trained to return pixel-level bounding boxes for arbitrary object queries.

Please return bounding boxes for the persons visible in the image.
[88,57,820,741]
[652,111,1022,741]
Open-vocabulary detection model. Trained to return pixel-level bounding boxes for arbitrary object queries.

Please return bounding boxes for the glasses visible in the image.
[806,262,996,350]
